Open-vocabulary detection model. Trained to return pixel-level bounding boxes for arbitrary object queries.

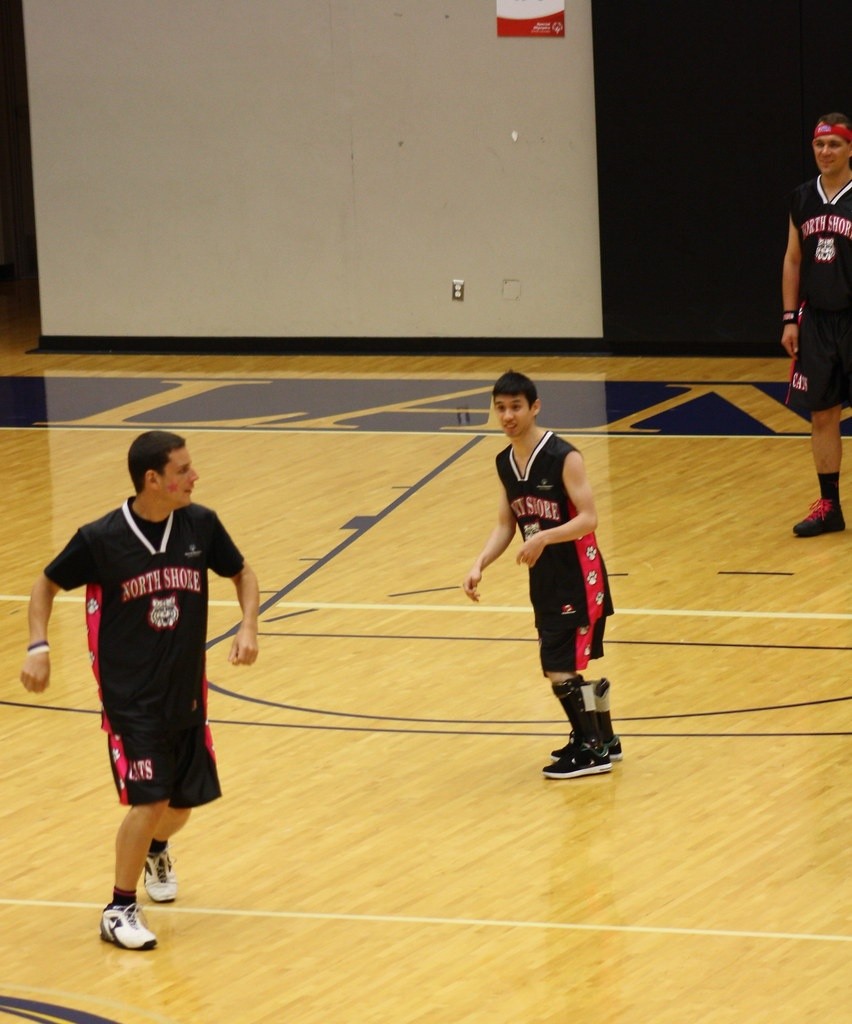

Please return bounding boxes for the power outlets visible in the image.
[502,280,521,301]
[452,280,465,301]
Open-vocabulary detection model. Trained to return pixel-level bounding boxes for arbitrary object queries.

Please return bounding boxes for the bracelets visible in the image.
[27,641,50,656]
[782,310,798,323]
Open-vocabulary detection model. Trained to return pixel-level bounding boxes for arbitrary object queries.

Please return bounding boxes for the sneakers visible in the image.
[99,901,162,947]
[542,736,614,780]
[549,730,628,760]
[792,499,848,534]
[144,845,179,901]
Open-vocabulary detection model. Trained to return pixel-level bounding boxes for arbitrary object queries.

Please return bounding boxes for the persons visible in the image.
[21,431,259,950]
[462,368,623,780]
[781,111,852,538]
